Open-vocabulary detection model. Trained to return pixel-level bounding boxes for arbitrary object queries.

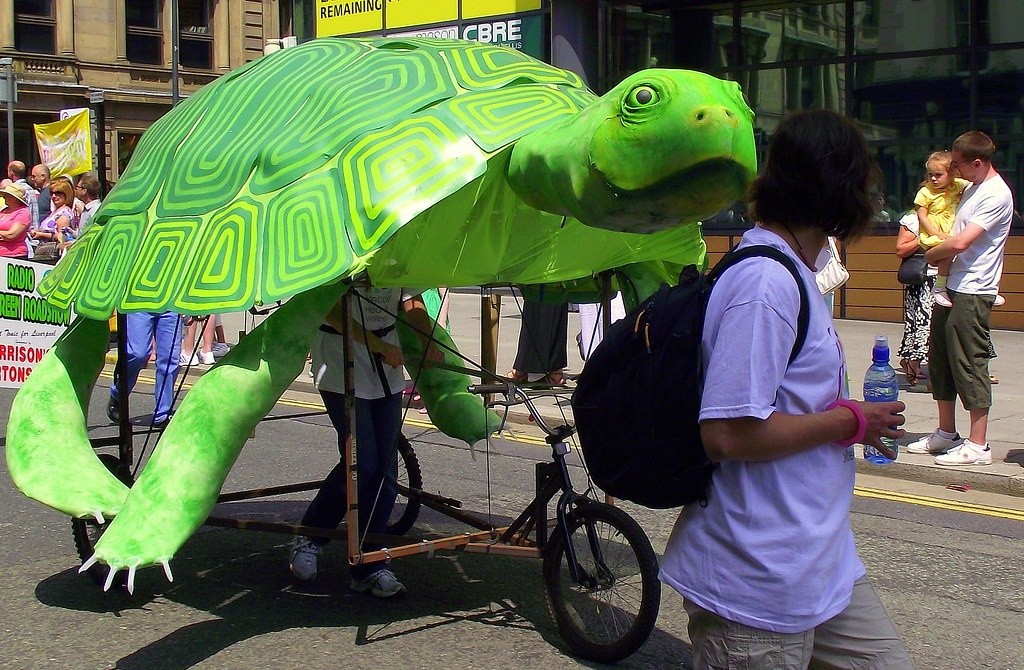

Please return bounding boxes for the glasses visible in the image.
[74,185,83,189]
[949,156,983,172]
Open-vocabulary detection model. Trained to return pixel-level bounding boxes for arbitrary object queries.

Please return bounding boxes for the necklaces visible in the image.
[785,226,820,272]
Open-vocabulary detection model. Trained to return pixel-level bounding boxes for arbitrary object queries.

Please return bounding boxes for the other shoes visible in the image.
[107,394,120,425]
[154,415,171,428]
[988,373,998,384]
[402,383,418,396]
[419,406,428,414]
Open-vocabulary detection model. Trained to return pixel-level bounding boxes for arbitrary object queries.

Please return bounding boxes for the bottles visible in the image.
[61,227,73,250]
[862,335,899,464]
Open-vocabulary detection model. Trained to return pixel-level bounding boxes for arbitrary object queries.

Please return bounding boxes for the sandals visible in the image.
[911,360,927,380]
[494,367,528,386]
[900,358,919,386]
[528,370,566,387]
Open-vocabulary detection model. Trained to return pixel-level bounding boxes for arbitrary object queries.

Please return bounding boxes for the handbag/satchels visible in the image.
[34,241,59,264]
[815,236,850,296]
[896,253,928,284]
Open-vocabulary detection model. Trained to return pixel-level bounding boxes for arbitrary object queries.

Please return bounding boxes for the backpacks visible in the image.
[570,242,811,510]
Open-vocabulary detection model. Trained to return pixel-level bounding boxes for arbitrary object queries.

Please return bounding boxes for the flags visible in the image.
[34,110,92,182]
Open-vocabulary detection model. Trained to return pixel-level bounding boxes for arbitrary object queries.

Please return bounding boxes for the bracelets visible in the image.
[823,398,867,449]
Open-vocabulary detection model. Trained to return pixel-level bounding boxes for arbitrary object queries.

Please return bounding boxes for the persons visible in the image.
[894,130,1013,467]
[149,311,231,367]
[578,291,626,364]
[503,284,567,390]
[287,282,445,600]
[106,310,183,429]
[405,286,449,414]
[0,160,102,266]
[651,108,912,669]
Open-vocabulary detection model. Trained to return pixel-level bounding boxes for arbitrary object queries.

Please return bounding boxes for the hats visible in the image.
[54,174,75,192]
[1,184,29,207]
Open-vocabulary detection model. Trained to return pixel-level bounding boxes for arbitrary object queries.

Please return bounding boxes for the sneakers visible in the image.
[930,284,952,307]
[287,535,318,581]
[178,348,199,366]
[992,294,1005,306]
[368,569,406,599]
[197,348,215,365]
[212,341,231,357]
[906,430,964,455]
[934,438,992,466]
